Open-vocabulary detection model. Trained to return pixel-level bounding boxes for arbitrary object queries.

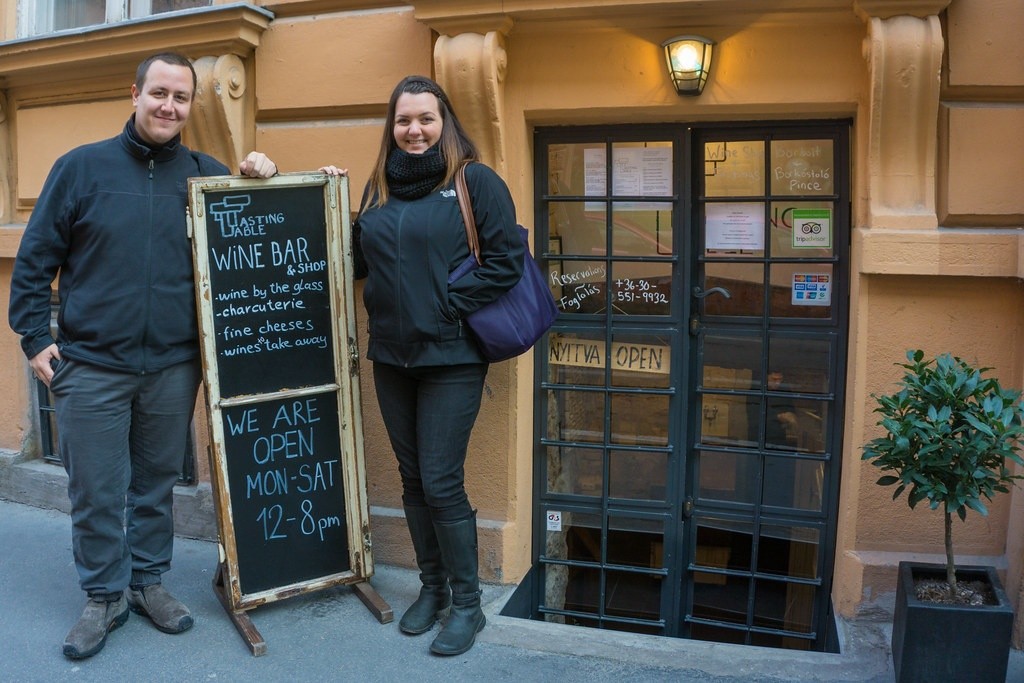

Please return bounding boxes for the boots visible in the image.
[398,573,452,634]
[429,588,486,655]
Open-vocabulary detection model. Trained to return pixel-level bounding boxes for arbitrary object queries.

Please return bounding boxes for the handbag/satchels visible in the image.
[451,224,561,364]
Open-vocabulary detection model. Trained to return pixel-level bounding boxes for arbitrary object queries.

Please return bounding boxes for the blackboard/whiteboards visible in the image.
[186,170,376,613]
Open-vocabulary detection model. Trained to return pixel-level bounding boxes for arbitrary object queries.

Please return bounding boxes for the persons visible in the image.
[8,53,278,661]
[318,76,525,656]
[746,365,797,443]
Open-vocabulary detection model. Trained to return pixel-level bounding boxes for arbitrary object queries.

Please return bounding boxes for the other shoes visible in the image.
[124,584,194,634]
[62,594,129,659]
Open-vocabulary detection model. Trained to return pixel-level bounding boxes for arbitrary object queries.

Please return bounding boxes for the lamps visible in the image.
[661,36,714,95]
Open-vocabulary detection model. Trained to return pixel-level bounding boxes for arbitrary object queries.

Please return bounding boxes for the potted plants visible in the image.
[861,348,1024,683]
